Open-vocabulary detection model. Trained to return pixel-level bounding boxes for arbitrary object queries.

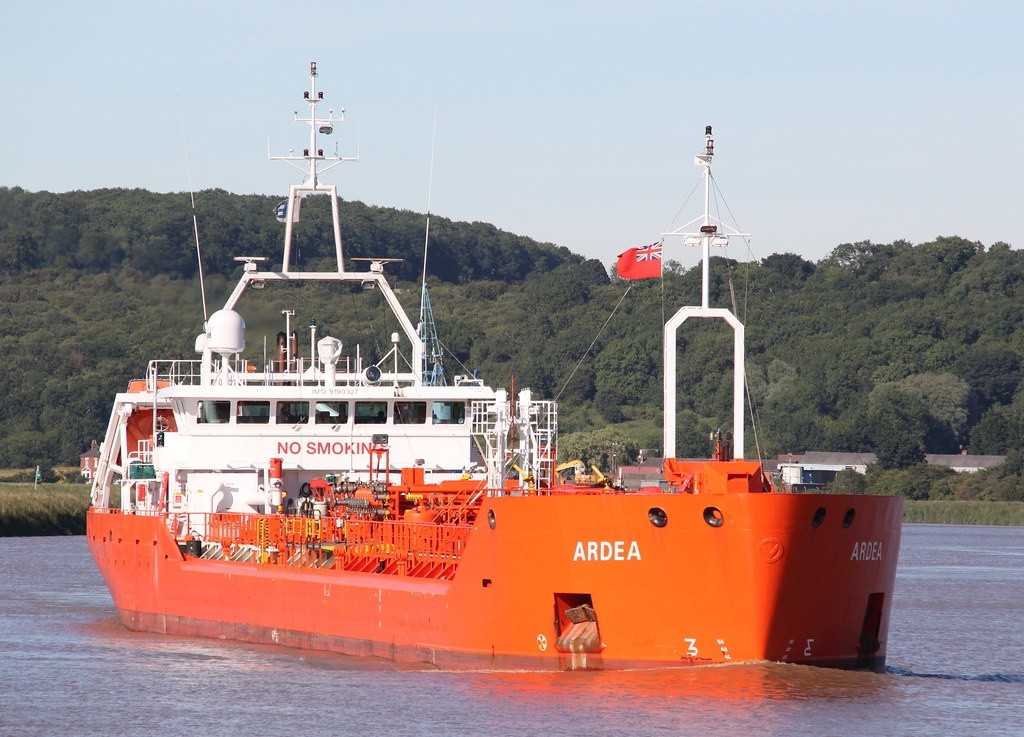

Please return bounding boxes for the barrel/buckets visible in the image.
[129,461,155,479]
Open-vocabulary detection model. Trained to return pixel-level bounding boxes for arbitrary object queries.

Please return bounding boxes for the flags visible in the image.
[617,242,662,280]
[273,197,300,223]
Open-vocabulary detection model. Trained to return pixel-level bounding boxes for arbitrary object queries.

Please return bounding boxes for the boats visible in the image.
[86,54,912,676]
